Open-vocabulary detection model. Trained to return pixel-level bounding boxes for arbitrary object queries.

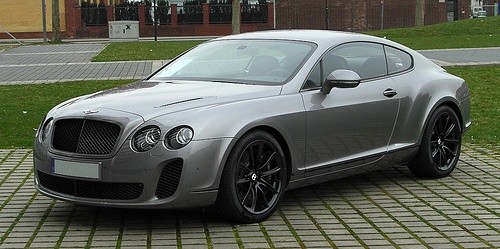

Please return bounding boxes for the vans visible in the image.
[473,6,487,17]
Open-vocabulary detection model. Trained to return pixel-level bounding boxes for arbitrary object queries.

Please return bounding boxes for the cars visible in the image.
[32,30,472,225]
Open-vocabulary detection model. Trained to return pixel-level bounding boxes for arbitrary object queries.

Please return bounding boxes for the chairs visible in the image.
[247,54,397,85]
